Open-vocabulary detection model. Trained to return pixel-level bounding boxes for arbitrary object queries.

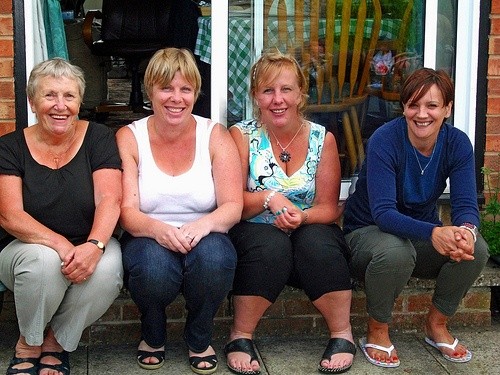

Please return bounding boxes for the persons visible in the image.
[225,53,358,375]
[343,66,491,368]
[0,56,124,375]
[116,47,245,375]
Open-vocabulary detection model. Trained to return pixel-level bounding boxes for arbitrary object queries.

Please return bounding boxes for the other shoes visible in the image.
[137,350,166,370]
[189,354,218,375]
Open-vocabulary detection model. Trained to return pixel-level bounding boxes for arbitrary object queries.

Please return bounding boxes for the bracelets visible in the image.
[301,211,308,225]
[262,192,277,209]
[458,222,478,244]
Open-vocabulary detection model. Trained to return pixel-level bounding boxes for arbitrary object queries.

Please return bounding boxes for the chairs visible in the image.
[263,0,414,175]
[82,0,199,115]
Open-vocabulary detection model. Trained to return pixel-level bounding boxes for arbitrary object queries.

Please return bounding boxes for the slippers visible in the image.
[224,338,262,375]
[424,334,472,363]
[7,352,40,375]
[358,336,400,368]
[318,337,356,374]
[38,350,70,375]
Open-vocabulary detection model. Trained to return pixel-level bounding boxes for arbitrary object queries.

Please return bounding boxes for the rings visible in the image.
[186,234,192,240]
[84,278,86,280]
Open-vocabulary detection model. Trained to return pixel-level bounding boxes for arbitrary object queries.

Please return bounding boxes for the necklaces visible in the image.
[410,134,438,175]
[271,121,303,162]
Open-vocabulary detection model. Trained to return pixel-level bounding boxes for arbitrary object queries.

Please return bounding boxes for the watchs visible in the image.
[87,239,106,254]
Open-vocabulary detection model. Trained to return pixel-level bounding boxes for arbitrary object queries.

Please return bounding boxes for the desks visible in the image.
[194,16,414,125]
